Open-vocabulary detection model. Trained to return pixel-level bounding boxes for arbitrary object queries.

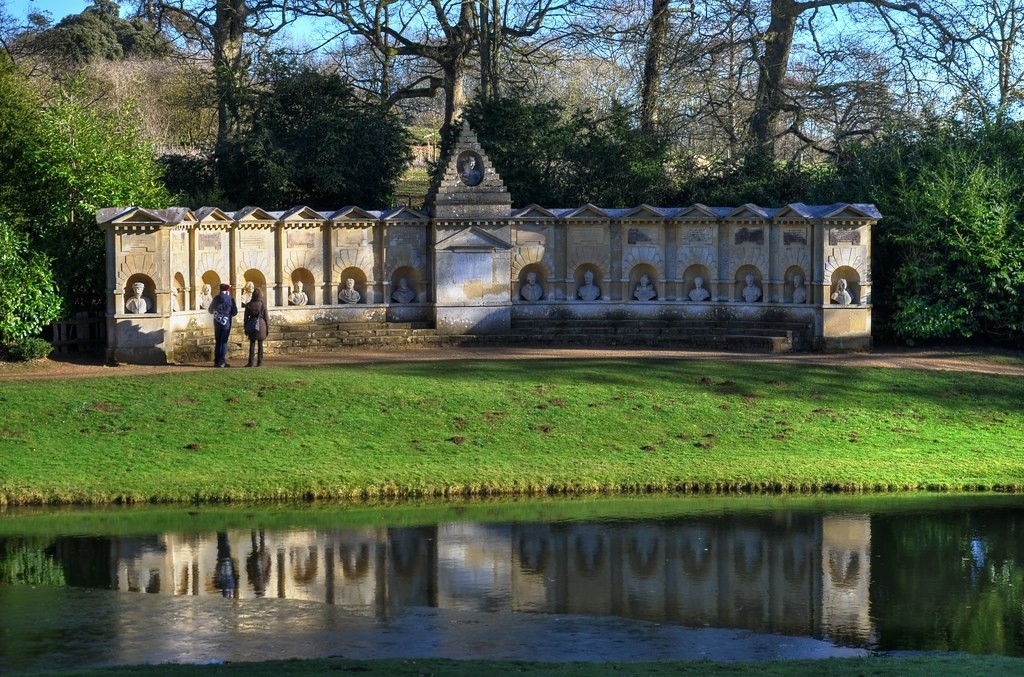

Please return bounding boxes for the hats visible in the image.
[219,283,230,290]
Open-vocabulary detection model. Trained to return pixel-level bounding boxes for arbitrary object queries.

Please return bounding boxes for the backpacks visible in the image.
[245,317,260,332]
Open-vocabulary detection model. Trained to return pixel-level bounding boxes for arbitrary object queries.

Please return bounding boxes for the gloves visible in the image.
[245,330,247,335]
[266,330,268,336]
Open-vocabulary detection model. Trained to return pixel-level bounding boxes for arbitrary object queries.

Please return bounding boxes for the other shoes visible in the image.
[214,362,230,367]
[244,363,253,366]
[255,364,260,367]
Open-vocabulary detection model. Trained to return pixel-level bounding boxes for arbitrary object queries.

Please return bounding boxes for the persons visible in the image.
[577,269,601,300]
[339,278,360,304]
[742,274,762,304]
[520,272,542,301]
[199,284,213,310]
[634,274,657,301]
[391,278,416,303]
[831,278,854,305]
[125,282,153,314]
[792,274,806,305]
[288,280,308,306]
[208,282,238,368]
[243,287,269,367]
[172,286,181,312]
[459,155,480,187]
[241,281,255,305]
[687,277,710,302]
